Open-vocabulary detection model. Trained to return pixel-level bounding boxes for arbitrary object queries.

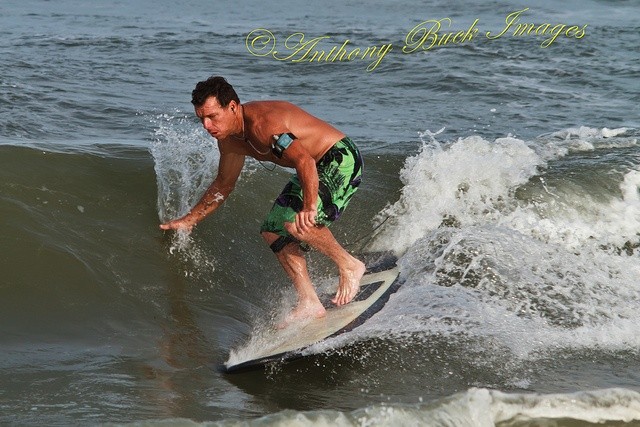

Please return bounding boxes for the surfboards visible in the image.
[223,249,407,373]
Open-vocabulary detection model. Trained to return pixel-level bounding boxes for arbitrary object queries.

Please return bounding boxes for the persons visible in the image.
[159,77,365,335]
